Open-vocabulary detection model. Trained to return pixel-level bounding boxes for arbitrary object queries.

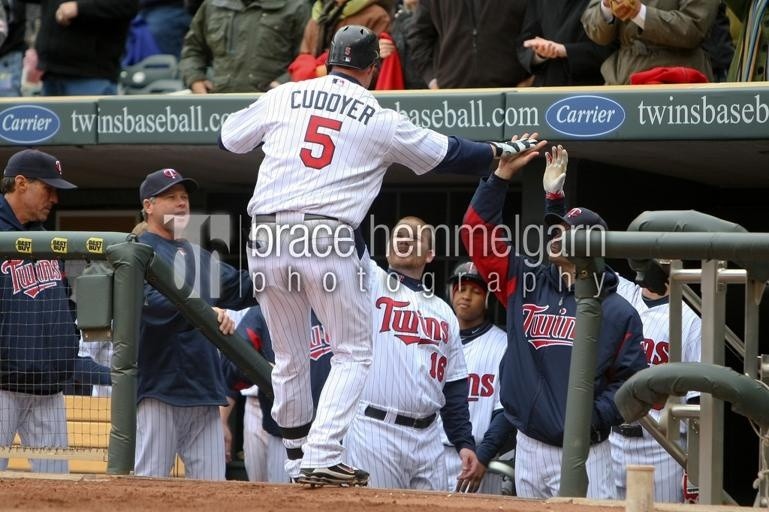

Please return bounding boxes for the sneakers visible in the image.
[297,460,369,488]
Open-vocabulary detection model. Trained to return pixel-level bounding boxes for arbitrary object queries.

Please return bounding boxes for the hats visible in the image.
[139,166,200,202]
[542,205,609,232]
[4,146,77,192]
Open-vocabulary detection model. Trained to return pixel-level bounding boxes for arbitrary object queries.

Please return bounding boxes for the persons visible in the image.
[208,299,335,485]
[135,169,260,480]
[462,131,646,501]
[440,260,517,497]
[0,149,78,475]
[541,144,703,505]
[341,213,478,495]
[218,26,540,483]
[1,1,769,98]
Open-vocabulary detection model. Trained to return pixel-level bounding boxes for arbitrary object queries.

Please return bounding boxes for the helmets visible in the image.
[326,25,382,71]
[445,260,489,290]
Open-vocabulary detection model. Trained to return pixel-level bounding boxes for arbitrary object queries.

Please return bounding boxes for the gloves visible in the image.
[540,145,570,197]
[492,133,537,168]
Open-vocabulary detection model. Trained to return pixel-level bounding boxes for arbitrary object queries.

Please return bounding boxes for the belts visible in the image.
[250,206,323,224]
[365,404,438,429]
[608,424,645,438]
